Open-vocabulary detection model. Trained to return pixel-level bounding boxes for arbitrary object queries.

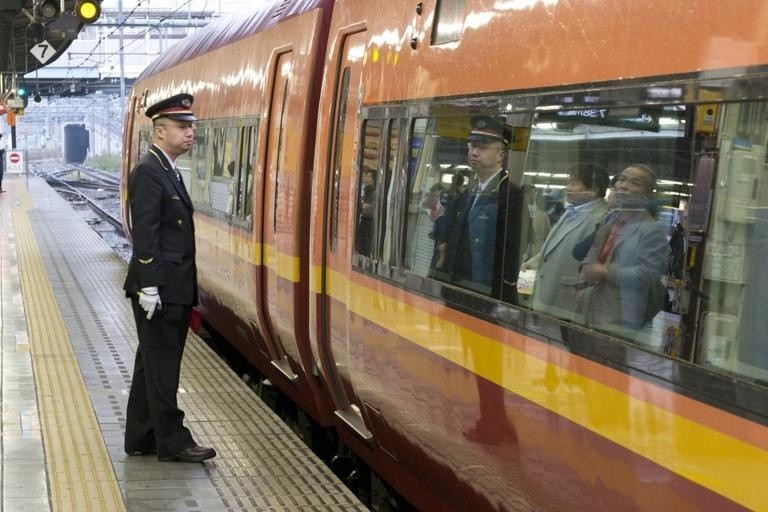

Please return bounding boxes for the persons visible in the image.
[572,165,670,331]
[123,93,216,463]
[0,133,6,193]
[357,170,376,258]
[528,163,608,321]
[227,160,253,216]
[446,116,530,445]
[669,223,684,282]
[422,171,567,284]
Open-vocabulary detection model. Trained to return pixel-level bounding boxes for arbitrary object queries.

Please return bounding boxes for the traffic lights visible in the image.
[32,0,62,25]
[76,1,101,23]
[18,86,28,108]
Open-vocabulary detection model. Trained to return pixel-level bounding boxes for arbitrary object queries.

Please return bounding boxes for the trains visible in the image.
[120,0,767,511]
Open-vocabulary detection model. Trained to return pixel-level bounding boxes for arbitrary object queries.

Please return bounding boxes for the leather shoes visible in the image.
[126,445,216,463]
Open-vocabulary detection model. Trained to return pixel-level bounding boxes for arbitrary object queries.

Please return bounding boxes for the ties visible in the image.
[469,185,482,213]
[174,169,180,182]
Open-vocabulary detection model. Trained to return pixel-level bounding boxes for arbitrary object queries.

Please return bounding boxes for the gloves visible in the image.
[138,286,162,320]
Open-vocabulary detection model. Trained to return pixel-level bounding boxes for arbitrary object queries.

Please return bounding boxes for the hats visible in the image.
[466,111,513,145]
[145,93,199,122]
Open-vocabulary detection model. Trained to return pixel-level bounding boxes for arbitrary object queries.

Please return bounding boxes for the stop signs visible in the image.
[9,153,20,163]
